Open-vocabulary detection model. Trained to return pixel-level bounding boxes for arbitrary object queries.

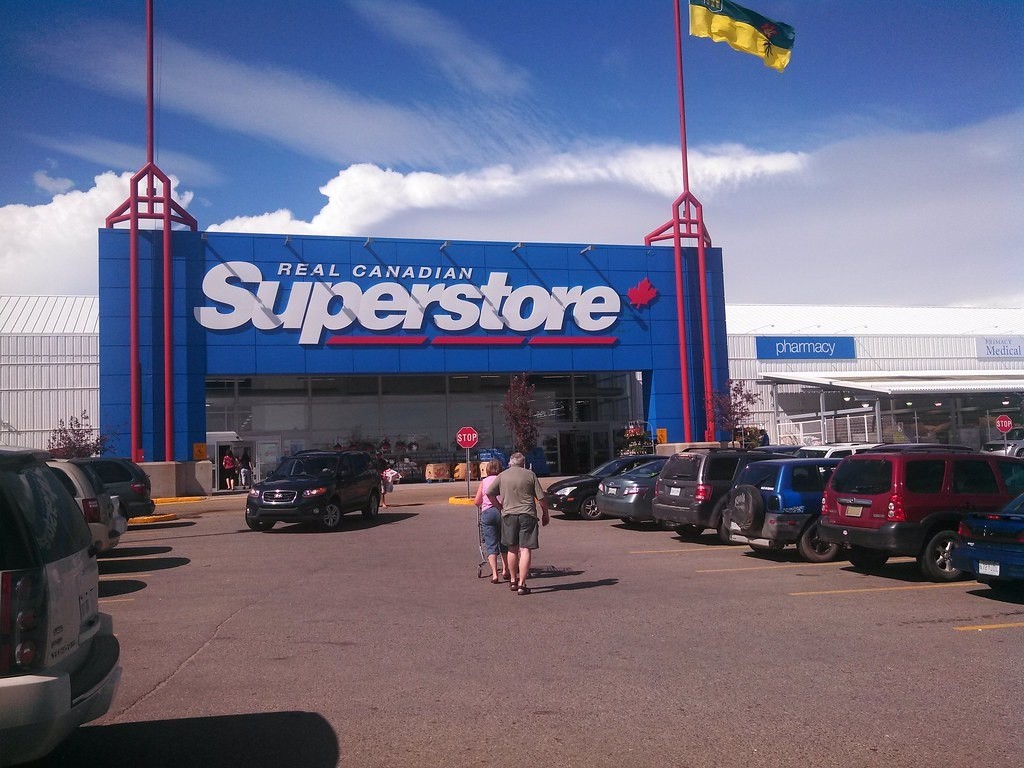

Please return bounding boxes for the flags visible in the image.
[689,0,796,73]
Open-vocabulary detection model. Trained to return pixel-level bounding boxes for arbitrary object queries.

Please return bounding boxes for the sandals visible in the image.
[509,582,518,591]
[517,586,531,595]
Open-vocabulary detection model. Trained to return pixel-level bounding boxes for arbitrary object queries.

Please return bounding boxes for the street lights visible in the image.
[637,421,654,454]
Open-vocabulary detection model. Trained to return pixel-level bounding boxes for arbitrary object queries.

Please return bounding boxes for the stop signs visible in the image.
[456,426,478,449]
[996,415,1012,433]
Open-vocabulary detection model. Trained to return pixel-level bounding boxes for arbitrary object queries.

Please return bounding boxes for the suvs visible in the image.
[0,445,122,768]
[80,457,156,522]
[722,458,845,562]
[651,447,790,540]
[816,442,1024,582]
[245,448,382,532]
[794,442,884,459]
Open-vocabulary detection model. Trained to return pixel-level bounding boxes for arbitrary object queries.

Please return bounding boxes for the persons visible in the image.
[734,435,743,448]
[373,451,393,509]
[485,452,549,595]
[223,450,251,490]
[474,461,510,584]
[758,430,769,446]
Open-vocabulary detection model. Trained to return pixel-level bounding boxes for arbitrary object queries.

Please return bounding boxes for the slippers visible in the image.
[502,573,510,580]
[491,579,498,584]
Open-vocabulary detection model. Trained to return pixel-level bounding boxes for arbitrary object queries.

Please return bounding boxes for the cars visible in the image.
[45,458,127,554]
[595,459,669,526]
[979,427,1024,459]
[751,446,802,455]
[544,455,670,520]
[951,494,1024,596]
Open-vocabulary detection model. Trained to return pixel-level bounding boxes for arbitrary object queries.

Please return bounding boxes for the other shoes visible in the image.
[249,487,251,489]
[232,489,234,490]
[383,505,387,508]
[243,486,245,489]
[227,488,230,489]
